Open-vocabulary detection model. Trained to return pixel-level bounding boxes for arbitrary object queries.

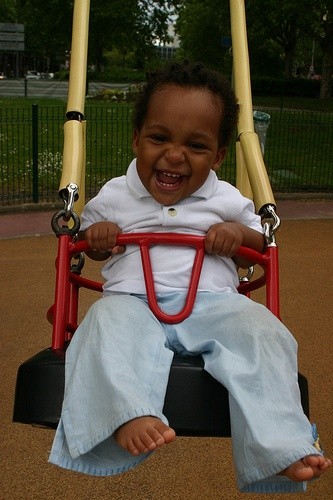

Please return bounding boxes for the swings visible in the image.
[10,1,312,439]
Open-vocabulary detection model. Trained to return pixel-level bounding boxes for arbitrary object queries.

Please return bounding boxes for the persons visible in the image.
[45,57,333,493]
